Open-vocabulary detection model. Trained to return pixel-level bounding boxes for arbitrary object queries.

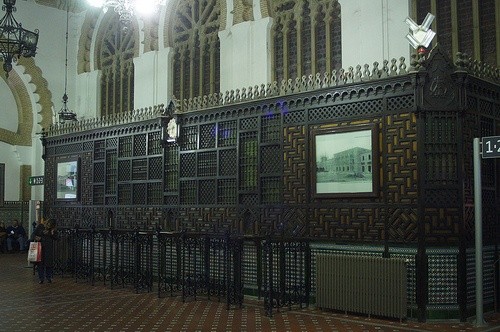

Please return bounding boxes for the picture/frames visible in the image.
[52,156,81,201]
[307,123,379,199]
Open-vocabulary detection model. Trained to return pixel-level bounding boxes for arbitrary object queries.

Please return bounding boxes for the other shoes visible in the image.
[39,278,52,284]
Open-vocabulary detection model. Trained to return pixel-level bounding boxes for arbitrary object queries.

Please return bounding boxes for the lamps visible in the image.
[57,5,76,124]
[0,0,39,79]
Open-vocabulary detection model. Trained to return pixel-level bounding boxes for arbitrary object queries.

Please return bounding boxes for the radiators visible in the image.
[315,253,407,321]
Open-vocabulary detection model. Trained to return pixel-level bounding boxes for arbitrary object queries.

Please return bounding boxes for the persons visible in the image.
[6,220,26,254]
[0,221,7,253]
[30,218,60,284]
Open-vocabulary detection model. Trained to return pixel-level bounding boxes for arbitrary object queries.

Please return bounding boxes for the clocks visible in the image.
[160,99,182,144]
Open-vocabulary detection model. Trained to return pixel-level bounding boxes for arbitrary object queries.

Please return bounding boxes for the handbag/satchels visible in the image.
[28,240,41,262]
